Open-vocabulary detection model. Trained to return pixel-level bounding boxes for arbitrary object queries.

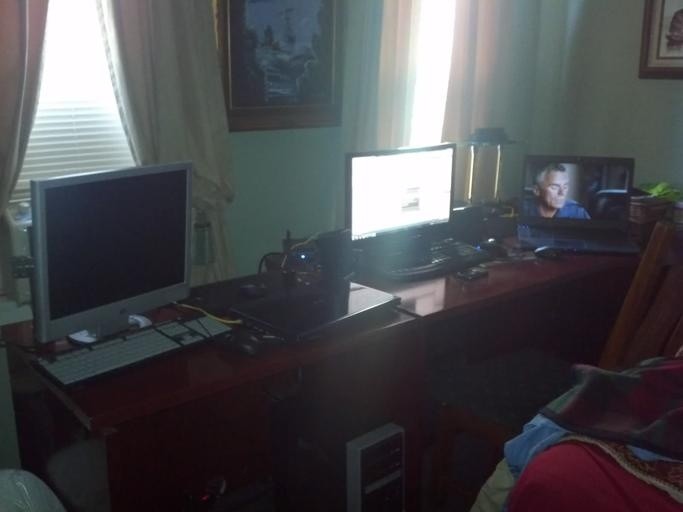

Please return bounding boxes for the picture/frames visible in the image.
[211,1,348,132]
[637,3,683,80]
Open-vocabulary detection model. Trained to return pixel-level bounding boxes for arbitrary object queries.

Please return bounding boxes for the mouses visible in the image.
[232,330,264,358]
[470,234,503,258]
[533,245,560,260]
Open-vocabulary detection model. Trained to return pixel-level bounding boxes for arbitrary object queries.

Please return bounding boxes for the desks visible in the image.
[348,195,683,438]
[0,271,421,511]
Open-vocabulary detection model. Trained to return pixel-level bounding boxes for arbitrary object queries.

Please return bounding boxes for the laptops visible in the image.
[516,154,641,254]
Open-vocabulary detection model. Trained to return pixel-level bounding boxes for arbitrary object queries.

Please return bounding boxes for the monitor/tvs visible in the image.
[344,142,457,246]
[30,160,194,345]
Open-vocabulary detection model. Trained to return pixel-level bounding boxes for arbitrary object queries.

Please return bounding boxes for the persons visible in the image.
[523,163,591,219]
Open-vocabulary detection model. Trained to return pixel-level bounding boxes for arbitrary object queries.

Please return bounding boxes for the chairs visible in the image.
[599,220,682,379]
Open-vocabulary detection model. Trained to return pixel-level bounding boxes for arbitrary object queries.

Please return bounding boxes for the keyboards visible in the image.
[373,238,491,282]
[30,319,206,391]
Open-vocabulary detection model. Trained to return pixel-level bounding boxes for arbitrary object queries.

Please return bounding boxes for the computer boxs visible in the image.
[268,394,407,512]
